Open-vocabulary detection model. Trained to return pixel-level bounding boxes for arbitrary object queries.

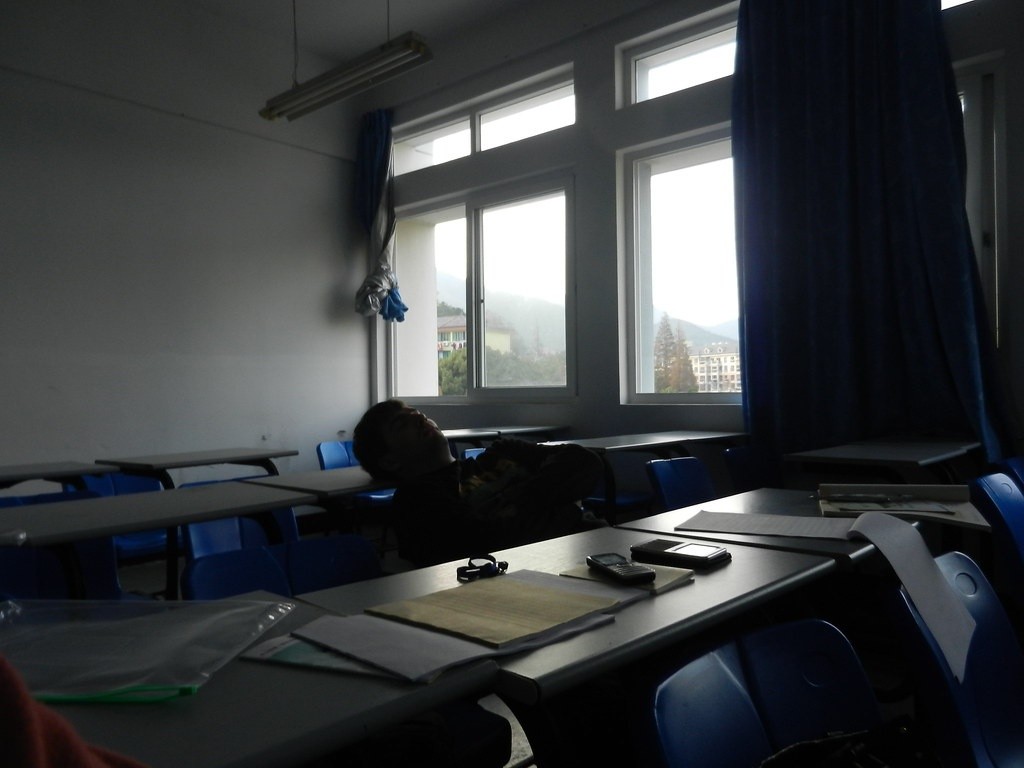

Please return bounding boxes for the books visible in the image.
[241,561,694,683]
[675,508,978,685]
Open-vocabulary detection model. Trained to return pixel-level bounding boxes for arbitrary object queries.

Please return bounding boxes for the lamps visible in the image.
[257,0,435,122]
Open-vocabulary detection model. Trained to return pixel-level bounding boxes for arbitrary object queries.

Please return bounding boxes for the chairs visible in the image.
[652,620,886,768]
[176,479,299,562]
[0,490,162,623]
[887,553,1024,768]
[316,441,396,508]
[645,455,721,511]
[178,534,512,768]
[723,446,792,492]
[80,473,168,556]
[962,469,1024,597]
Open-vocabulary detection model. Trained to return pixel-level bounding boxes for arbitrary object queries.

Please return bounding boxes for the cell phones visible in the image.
[585,553,655,585]
[630,539,725,560]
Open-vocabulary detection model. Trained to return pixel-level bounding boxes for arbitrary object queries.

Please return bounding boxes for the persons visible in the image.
[352,400,610,574]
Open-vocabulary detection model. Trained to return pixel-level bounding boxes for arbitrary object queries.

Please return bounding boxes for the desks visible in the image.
[783,434,982,550]
[441,429,499,456]
[0,460,119,491]
[94,447,300,490]
[243,465,371,498]
[6,589,498,768]
[463,425,569,442]
[294,526,841,768]
[613,487,925,572]
[0,481,319,602]
[537,430,747,497]
